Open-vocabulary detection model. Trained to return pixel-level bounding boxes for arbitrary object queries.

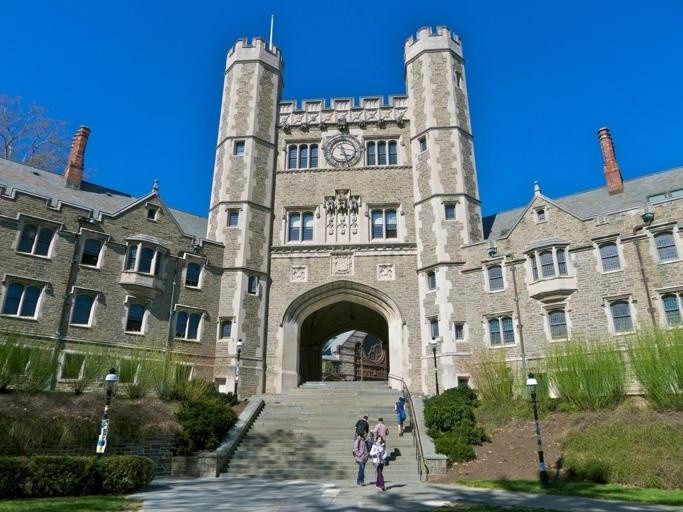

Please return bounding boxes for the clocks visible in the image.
[323,134,361,168]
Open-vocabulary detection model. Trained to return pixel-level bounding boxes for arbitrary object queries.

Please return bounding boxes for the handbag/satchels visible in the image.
[401,411,406,420]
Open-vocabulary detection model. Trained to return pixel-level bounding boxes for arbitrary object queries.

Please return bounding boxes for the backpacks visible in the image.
[352,440,360,458]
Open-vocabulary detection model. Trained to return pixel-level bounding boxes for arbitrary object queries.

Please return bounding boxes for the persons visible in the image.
[354,416,389,458]
[370,435,387,491]
[395,397,408,436]
[352,430,368,486]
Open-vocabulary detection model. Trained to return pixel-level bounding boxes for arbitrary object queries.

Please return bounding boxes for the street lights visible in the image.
[526,372,550,488]
[233,338,243,399]
[96,367,117,458]
[430,335,439,396]
